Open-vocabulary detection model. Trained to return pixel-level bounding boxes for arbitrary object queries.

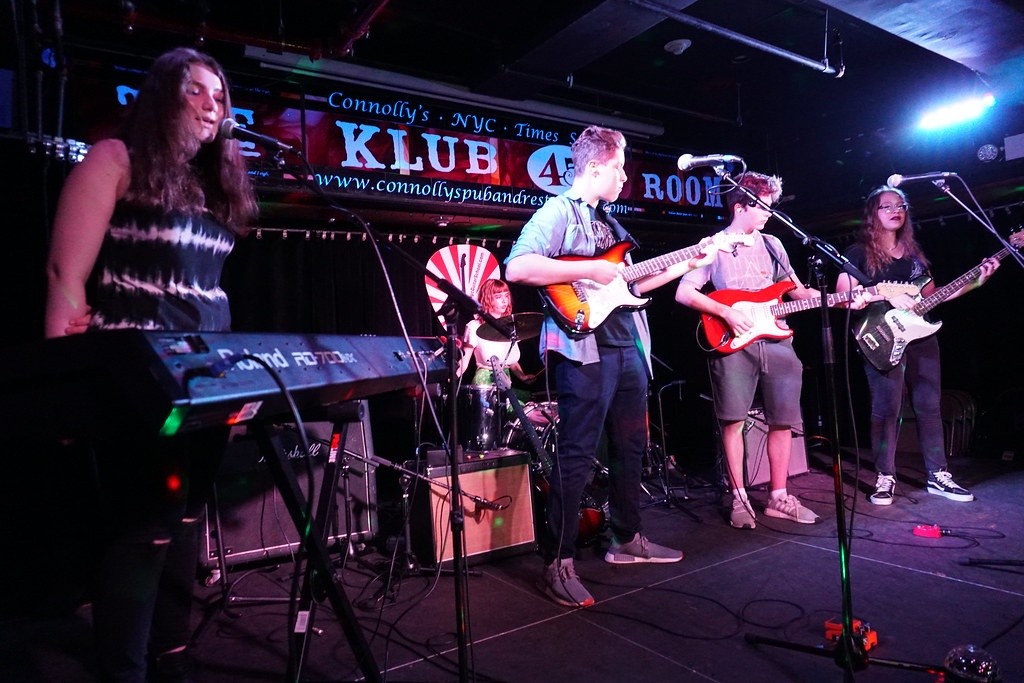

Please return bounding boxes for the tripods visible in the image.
[641,165,958,683]
[184,420,496,649]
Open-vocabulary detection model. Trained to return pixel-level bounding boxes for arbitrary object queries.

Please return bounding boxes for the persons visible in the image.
[505,125,717,609]
[836,188,1000,505]
[44,49,259,683]
[676,173,876,528]
[458,279,535,414]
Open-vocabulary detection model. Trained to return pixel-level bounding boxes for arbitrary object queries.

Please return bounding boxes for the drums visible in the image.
[430,384,503,455]
[504,401,609,510]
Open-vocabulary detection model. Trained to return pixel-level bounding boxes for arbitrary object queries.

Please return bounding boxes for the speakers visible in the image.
[792,431,806,439]
[407,446,538,574]
[198,400,378,569]
[745,407,809,487]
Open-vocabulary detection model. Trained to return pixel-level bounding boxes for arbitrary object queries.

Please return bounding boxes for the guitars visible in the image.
[849,223,1024,374]
[701,280,920,354]
[535,231,756,340]
[486,354,606,545]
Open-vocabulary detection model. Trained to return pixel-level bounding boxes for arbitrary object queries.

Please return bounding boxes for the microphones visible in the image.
[218,118,301,157]
[677,154,743,172]
[887,173,958,187]
[475,502,502,511]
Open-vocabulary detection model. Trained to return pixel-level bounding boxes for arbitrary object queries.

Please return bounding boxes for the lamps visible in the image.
[976,132,1024,164]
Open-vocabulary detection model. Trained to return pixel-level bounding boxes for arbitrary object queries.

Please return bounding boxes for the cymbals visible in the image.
[476,312,546,342]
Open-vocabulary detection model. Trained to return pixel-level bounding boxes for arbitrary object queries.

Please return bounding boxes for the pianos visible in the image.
[0,328,454,682]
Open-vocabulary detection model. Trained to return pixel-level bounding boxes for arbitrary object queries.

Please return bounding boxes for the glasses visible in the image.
[877,204,909,212]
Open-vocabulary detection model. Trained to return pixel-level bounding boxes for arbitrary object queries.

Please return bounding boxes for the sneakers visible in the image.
[764,494,823,524]
[535,565,594,607]
[604,533,683,564]
[869,472,896,506]
[730,498,756,529]
[927,470,974,502]
